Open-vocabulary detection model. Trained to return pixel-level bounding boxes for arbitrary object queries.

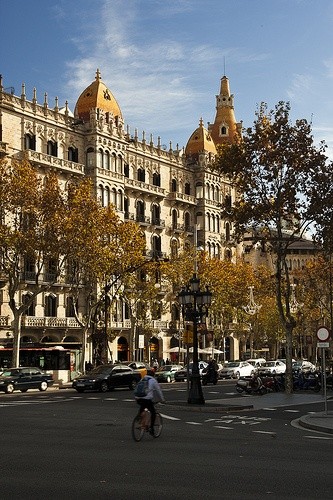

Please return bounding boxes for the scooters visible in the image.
[200,364,219,386]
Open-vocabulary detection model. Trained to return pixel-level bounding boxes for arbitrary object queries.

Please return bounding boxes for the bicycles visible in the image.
[132,401,166,442]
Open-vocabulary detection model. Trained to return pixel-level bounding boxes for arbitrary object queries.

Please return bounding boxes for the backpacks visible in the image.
[134,377,154,397]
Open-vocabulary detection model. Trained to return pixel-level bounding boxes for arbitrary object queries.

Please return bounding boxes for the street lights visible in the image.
[175,272,213,406]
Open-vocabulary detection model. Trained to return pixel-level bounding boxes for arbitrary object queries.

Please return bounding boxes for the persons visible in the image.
[134,368,166,434]
[206,359,220,380]
[153,359,177,371]
[86,361,95,372]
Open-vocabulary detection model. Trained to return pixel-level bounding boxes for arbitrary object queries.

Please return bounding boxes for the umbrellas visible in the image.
[162,345,224,355]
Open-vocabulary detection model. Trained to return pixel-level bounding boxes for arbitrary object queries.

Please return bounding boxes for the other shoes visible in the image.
[150,432,155,435]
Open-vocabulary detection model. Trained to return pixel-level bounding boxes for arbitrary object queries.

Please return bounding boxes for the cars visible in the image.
[0,367,55,394]
[121,357,333,395]
[72,364,141,393]
